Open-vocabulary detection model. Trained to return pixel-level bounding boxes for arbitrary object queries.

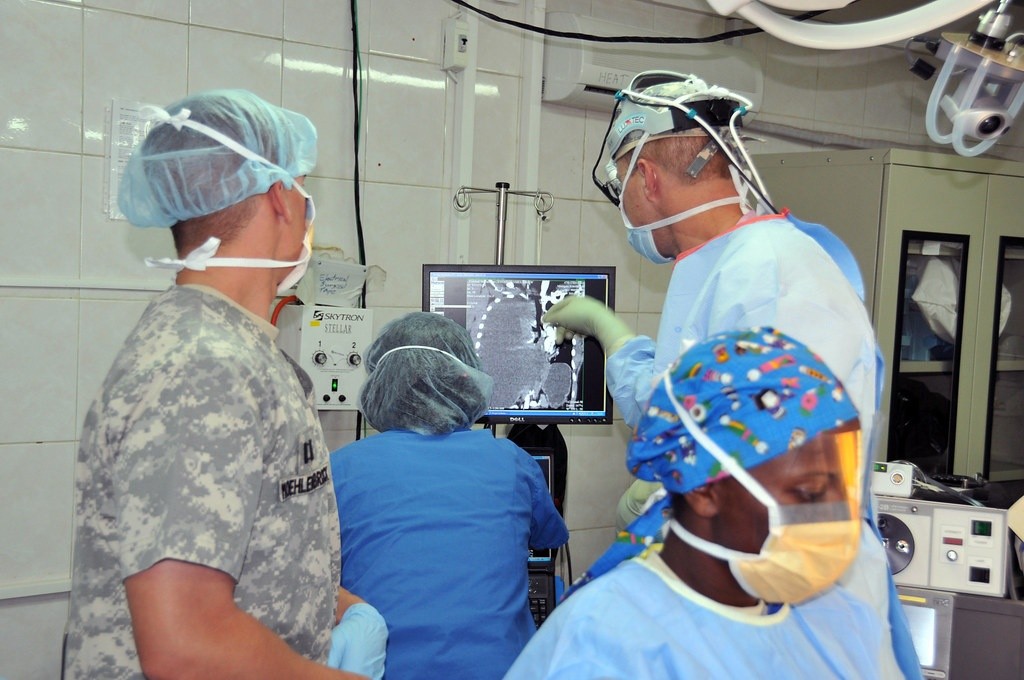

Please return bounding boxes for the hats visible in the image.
[556,327,860,603]
[614,81,768,178]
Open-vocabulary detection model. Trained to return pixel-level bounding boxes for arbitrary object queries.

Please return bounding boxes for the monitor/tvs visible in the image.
[422,263,618,425]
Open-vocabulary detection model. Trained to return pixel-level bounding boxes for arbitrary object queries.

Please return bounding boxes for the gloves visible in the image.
[542,294,635,358]
[328,604,389,680]
[616,478,664,536]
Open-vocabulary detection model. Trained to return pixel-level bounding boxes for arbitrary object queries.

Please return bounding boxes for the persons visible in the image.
[498,325,906,680]
[330,311,570,680]
[544,70,922,679]
[64,90,390,678]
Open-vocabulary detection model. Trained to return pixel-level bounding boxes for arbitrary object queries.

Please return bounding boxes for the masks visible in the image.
[618,73,753,264]
[640,337,859,605]
[141,104,317,294]
